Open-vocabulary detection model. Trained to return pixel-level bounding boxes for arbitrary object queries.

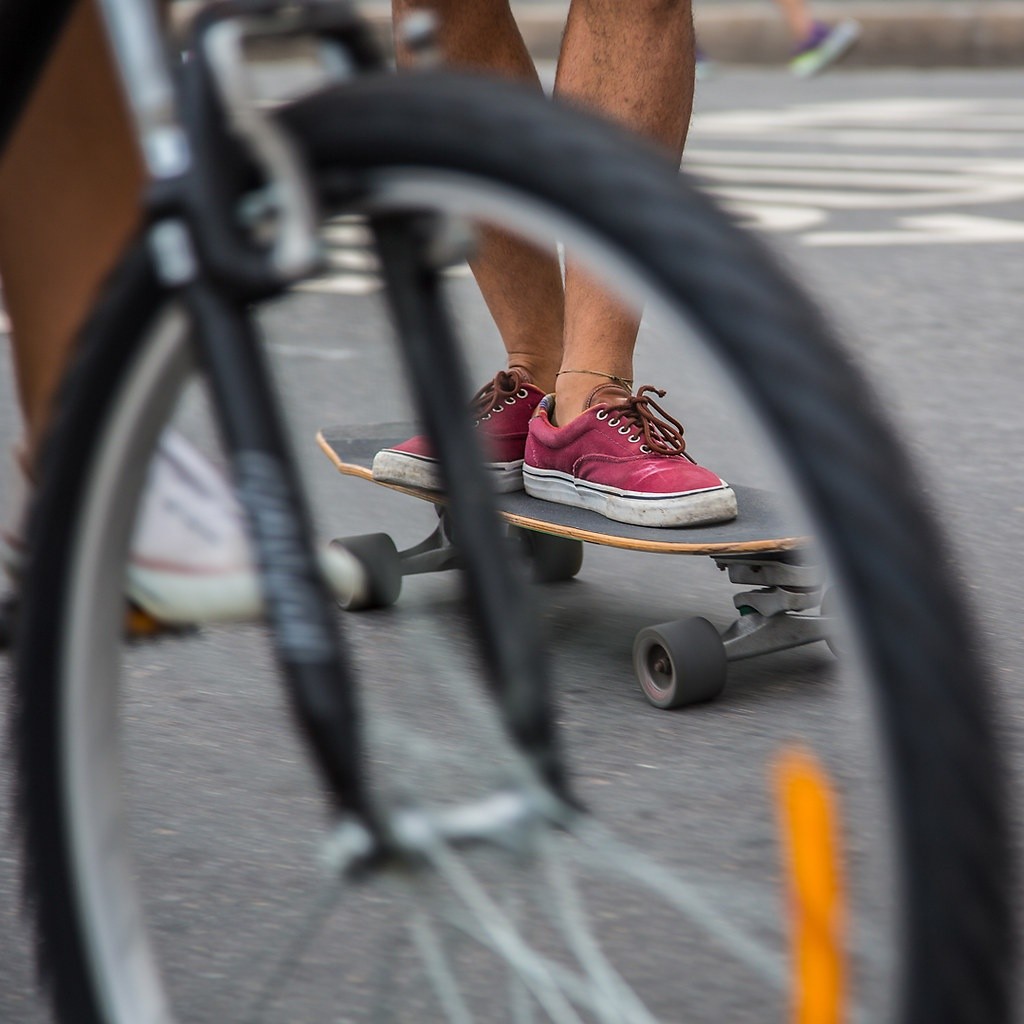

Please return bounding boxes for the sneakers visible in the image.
[371,370,547,497]
[0,426,370,625]
[520,383,739,530]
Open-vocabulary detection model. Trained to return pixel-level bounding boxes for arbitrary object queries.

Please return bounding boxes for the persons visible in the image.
[373,1,737,530]
[689,2,863,82]
[0,0,362,618]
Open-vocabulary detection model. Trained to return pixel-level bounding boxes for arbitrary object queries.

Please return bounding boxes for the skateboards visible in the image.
[309,410,861,711]
